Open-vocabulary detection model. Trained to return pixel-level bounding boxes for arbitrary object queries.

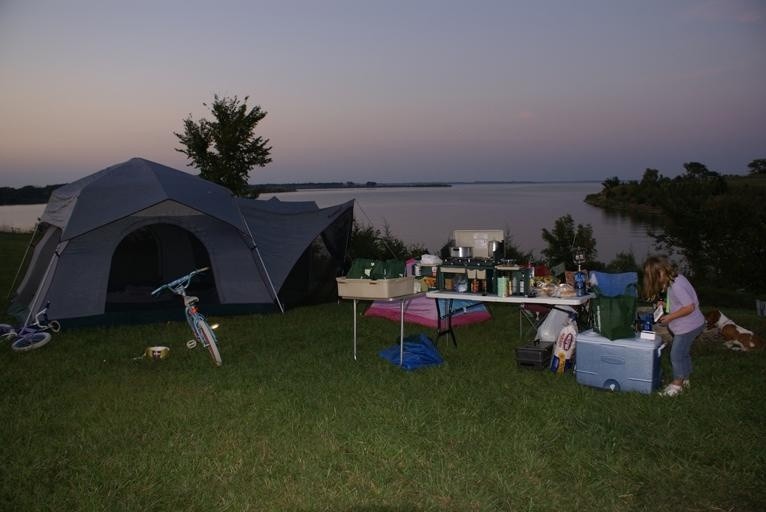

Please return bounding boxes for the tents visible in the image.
[6,157,357,327]
[362,291,495,330]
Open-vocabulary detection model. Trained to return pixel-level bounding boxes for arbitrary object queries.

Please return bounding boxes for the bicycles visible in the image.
[0,300,61,353]
[151,267,223,368]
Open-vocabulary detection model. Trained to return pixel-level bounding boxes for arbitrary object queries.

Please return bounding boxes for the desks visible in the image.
[341,289,595,368]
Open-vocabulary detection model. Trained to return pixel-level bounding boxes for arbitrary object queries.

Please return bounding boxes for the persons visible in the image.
[642,254,705,398]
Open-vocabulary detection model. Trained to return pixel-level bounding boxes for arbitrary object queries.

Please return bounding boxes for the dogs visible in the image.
[702,309,766,352]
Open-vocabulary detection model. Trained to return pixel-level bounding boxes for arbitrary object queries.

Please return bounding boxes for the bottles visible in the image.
[513,272,524,294]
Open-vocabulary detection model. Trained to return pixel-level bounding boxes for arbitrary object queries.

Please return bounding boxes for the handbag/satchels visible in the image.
[588,282,639,341]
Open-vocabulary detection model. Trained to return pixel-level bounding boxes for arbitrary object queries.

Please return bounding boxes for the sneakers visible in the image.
[682,380,691,390]
[657,383,683,397]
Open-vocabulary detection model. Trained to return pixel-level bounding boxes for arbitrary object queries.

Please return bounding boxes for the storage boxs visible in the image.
[573,327,667,395]
